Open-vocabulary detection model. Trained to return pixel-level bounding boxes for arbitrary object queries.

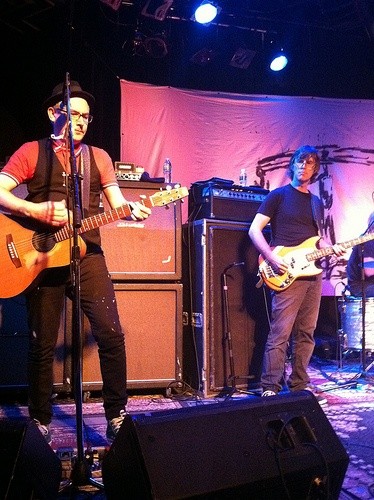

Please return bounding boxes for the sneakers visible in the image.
[34,418,52,444]
[301,386,328,405]
[105,410,126,439]
[261,390,276,398]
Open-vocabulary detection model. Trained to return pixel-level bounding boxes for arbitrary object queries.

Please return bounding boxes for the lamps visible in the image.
[101,0,289,72]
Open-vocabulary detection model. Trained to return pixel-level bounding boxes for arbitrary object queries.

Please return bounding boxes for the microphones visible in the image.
[341,281,358,298]
[62,72,70,104]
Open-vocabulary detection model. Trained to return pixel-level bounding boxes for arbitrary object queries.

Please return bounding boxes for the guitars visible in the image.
[0,182,191,299]
[258,232,374,291]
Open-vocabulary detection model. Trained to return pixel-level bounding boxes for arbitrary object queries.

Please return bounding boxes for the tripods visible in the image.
[215,261,259,401]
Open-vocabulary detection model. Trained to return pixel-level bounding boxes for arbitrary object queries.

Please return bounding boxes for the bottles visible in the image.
[163,157,172,182]
[239,169,248,187]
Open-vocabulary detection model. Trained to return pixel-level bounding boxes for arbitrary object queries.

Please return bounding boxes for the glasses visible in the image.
[56,108,93,123]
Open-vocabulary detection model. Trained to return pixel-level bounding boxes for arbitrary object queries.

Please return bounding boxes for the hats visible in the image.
[46,81,94,108]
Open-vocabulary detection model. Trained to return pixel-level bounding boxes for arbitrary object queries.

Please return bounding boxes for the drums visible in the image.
[338,296,374,353]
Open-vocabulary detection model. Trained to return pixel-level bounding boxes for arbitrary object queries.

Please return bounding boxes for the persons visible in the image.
[0,81,152,446]
[346,213,374,297]
[248,145,346,397]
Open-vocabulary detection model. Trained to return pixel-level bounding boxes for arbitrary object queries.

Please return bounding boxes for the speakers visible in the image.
[0,178,274,391]
[103,389,350,500]
[310,296,343,361]
[0,414,62,500]
